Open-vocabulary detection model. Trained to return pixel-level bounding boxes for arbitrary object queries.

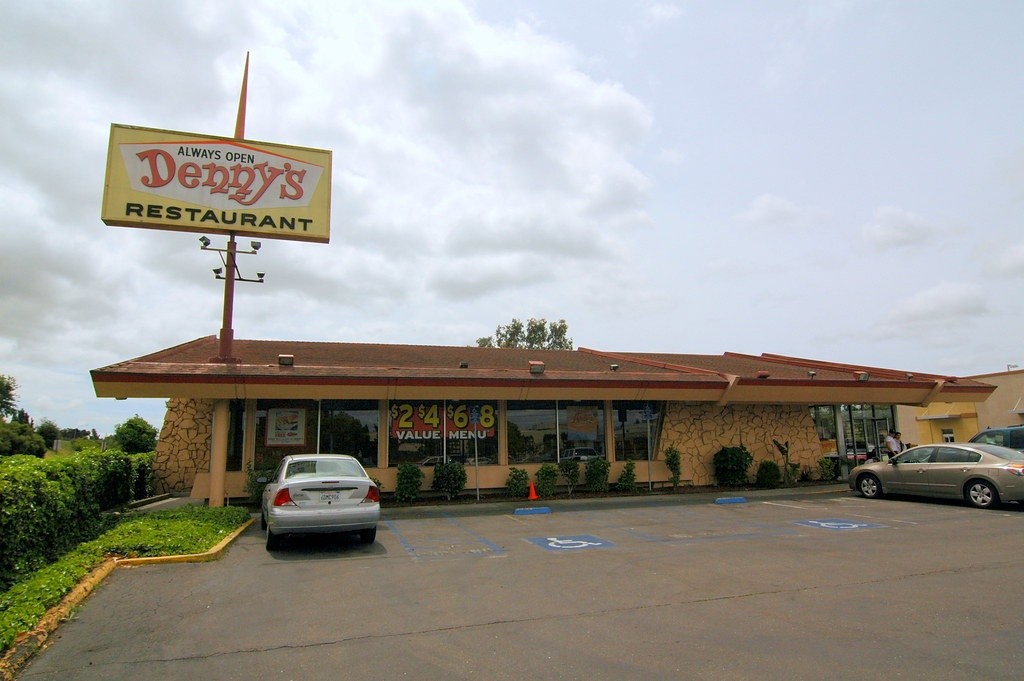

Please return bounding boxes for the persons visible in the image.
[893,432,904,452]
[886,429,898,459]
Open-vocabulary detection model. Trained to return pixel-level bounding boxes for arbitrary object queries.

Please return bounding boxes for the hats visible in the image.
[889,428,897,434]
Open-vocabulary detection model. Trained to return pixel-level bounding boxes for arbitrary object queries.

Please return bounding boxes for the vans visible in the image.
[966,424,1024,453]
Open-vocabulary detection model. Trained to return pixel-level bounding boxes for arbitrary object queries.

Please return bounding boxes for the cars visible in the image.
[848,441,1024,510]
[255,453,381,550]
